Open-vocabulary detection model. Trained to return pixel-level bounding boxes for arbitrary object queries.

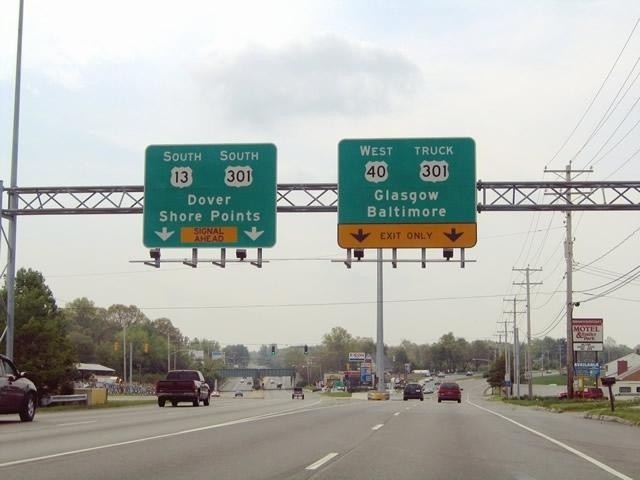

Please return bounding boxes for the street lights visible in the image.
[167,333,190,371]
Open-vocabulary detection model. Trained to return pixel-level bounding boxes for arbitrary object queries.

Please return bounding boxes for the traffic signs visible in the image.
[142,143,277,248]
[336,136,478,249]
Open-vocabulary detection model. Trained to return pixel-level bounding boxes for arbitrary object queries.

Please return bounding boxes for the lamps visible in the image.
[443,248,453,260]
[150,249,160,260]
[236,249,246,260]
[354,249,364,260]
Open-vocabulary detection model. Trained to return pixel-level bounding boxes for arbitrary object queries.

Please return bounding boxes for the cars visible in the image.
[403,384,423,401]
[292,387,304,400]
[438,383,463,403]
[235,390,243,397]
[0,353,38,421]
[212,390,220,397]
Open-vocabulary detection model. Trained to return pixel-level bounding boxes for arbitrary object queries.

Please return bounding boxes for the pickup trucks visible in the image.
[156,369,210,407]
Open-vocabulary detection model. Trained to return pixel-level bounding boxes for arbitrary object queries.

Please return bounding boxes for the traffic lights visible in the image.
[304,346,308,355]
[271,346,275,355]
